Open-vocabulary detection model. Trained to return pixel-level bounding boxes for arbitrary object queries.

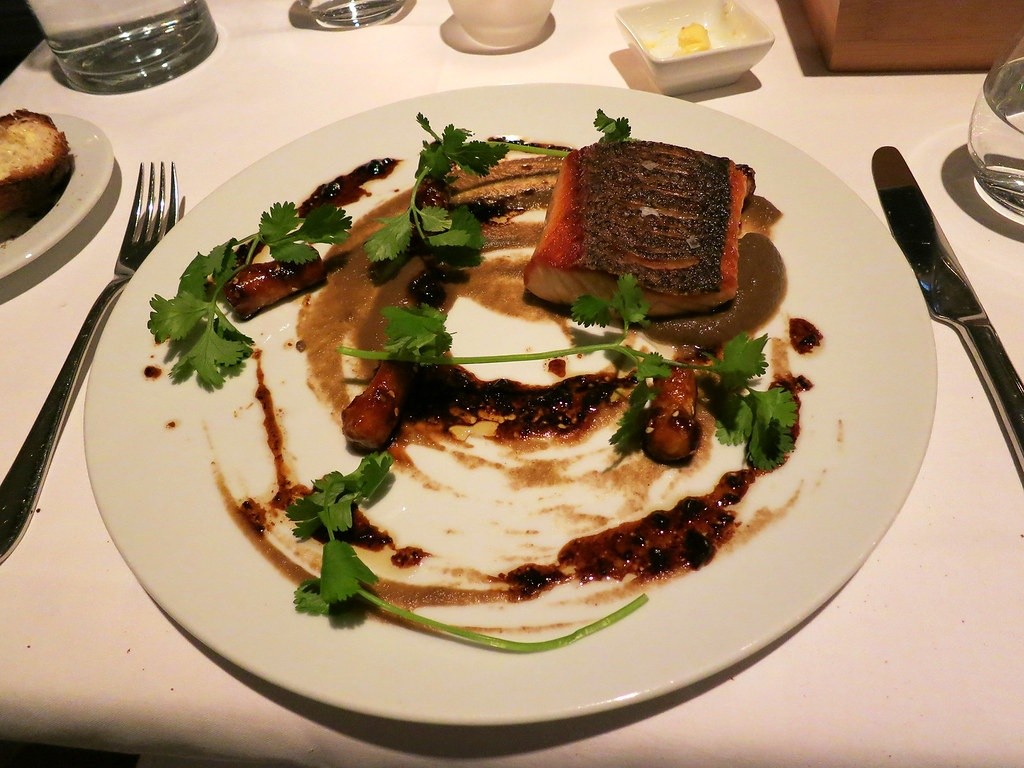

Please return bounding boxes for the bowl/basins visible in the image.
[615,0,776,96]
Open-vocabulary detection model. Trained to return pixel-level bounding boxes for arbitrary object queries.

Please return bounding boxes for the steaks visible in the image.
[521,139,747,319]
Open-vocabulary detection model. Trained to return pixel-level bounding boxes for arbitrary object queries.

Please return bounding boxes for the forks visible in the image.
[1,159,177,567]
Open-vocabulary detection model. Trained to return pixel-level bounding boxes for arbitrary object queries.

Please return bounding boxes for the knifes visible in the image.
[870,145,1023,477]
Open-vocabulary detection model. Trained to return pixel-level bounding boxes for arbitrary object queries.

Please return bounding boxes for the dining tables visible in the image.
[1,0,1021,765]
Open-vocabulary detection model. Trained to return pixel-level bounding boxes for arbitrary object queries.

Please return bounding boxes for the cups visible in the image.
[27,0,222,97]
[448,0,555,47]
[964,23,1023,214]
[295,0,404,30]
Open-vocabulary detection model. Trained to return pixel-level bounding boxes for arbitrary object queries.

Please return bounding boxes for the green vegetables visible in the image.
[335,273,796,470]
[146,93,631,388]
[284,451,648,652]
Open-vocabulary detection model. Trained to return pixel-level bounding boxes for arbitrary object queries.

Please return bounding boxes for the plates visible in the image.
[80,83,936,727]
[0,111,113,285]
